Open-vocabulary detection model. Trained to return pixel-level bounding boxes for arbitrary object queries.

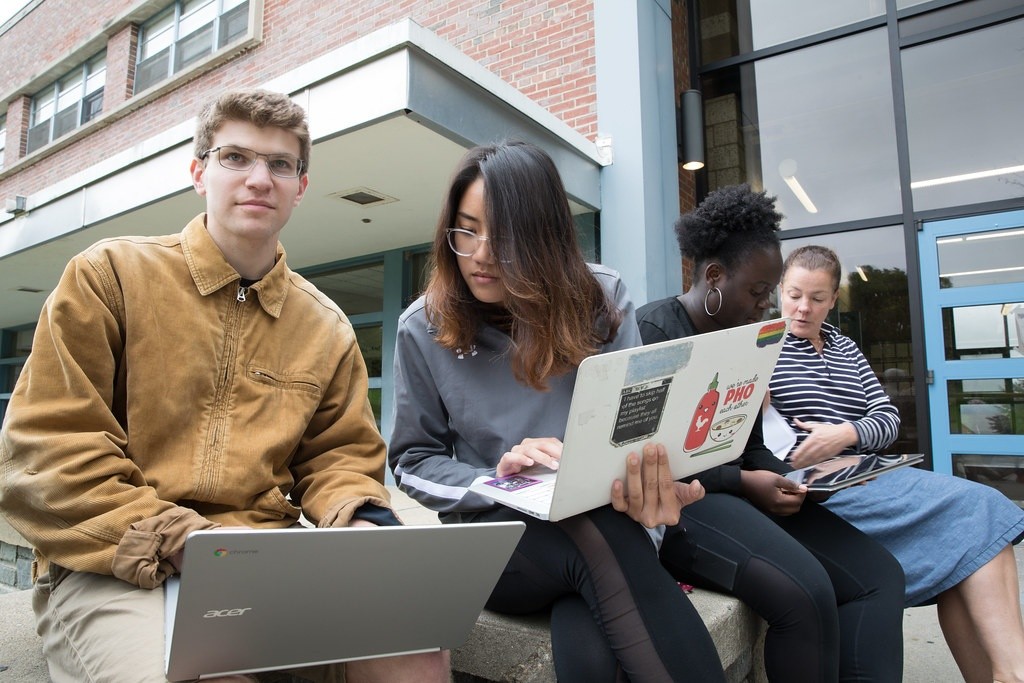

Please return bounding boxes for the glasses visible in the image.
[201,145,304,178]
[444,227,515,263]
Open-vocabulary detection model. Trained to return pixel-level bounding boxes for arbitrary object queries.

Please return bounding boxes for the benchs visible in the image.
[449,576,771,683]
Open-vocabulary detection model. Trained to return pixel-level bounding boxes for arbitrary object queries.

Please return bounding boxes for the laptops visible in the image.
[162,521,526,682]
[481,316,790,523]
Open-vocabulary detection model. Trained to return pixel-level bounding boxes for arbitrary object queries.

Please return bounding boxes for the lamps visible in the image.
[681,88,706,171]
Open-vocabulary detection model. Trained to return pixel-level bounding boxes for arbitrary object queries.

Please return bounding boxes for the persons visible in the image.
[388,135,729,683]
[0,90,453,683]
[634,179,1024,683]
